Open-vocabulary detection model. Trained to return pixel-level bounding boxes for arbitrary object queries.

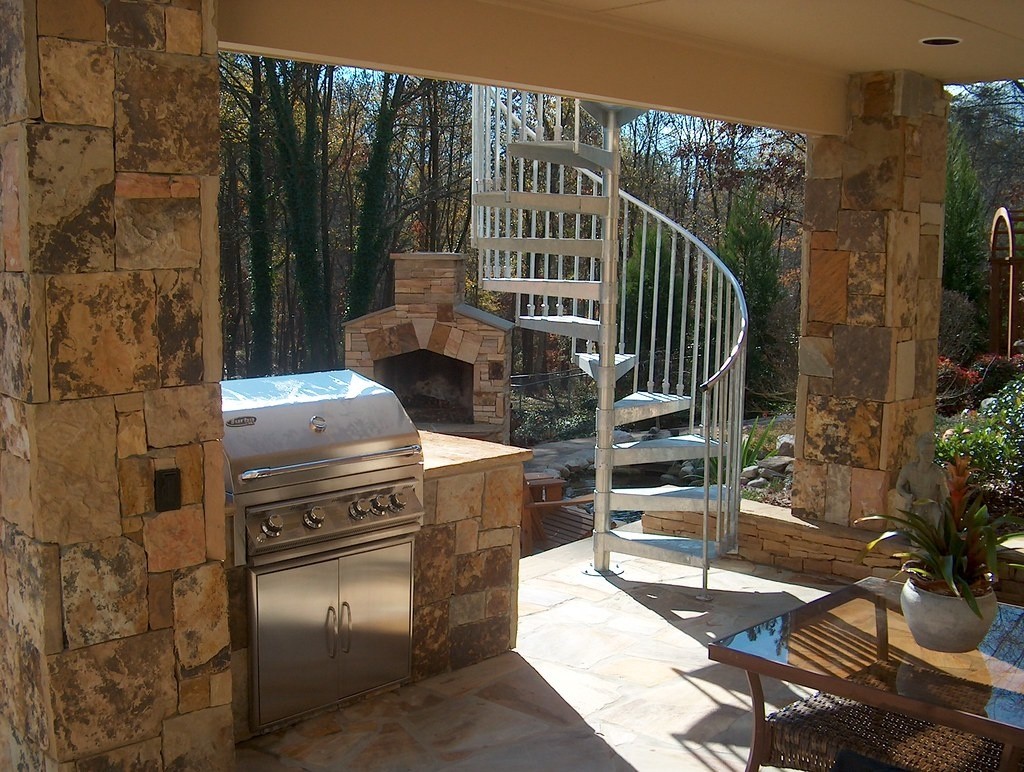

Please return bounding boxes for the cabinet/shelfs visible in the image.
[244,532,415,735]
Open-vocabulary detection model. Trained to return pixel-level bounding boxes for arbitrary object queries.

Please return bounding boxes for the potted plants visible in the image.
[851,450,1023,654]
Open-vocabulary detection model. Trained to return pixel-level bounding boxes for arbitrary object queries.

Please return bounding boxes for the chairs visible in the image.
[520,474,617,558]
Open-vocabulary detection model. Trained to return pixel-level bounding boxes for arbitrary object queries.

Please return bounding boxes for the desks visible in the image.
[708,576,1024,771]
